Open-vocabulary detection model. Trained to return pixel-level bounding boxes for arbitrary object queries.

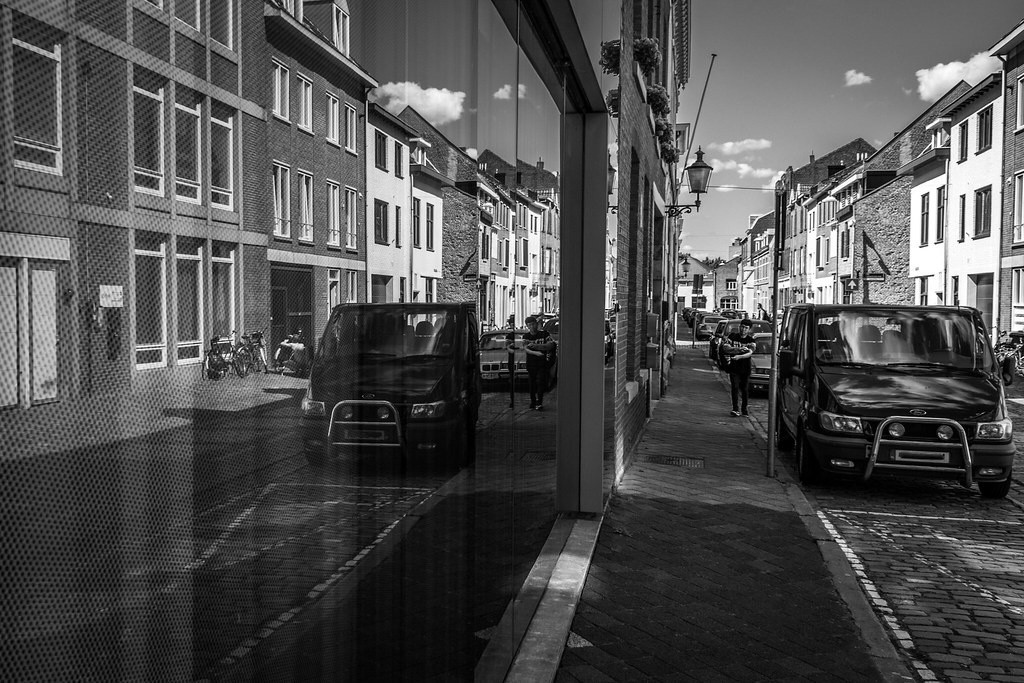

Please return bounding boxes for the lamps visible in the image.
[665,145,713,217]
[678,256,691,281]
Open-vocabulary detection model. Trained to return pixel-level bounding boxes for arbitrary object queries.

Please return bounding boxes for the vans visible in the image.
[299,300,484,470]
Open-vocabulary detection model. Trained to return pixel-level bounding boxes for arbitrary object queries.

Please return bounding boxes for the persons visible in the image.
[522,317,557,414]
[721,318,757,417]
[281,335,307,377]
[756,302,769,320]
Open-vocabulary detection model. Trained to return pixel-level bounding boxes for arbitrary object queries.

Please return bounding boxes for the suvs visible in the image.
[780,303,1019,498]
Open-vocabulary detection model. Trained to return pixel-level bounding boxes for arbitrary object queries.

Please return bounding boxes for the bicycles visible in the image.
[987,325,1024,380]
[204,322,295,378]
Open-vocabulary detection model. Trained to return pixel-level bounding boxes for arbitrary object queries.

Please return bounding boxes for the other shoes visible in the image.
[536,403,542,410]
[742,415,749,417]
[529,406,534,410]
[730,410,740,416]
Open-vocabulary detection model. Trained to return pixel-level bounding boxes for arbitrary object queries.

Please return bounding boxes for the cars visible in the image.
[478,330,538,388]
[714,320,774,372]
[532,308,618,365]
[682,307,750,361]
[746,332,782,399]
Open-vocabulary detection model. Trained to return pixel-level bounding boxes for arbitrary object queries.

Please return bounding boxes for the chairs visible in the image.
[756,341,773,354]
[415,321,434,352]
[753,326,763,333]
[731,327,739,333]
[815,321,925,361]
